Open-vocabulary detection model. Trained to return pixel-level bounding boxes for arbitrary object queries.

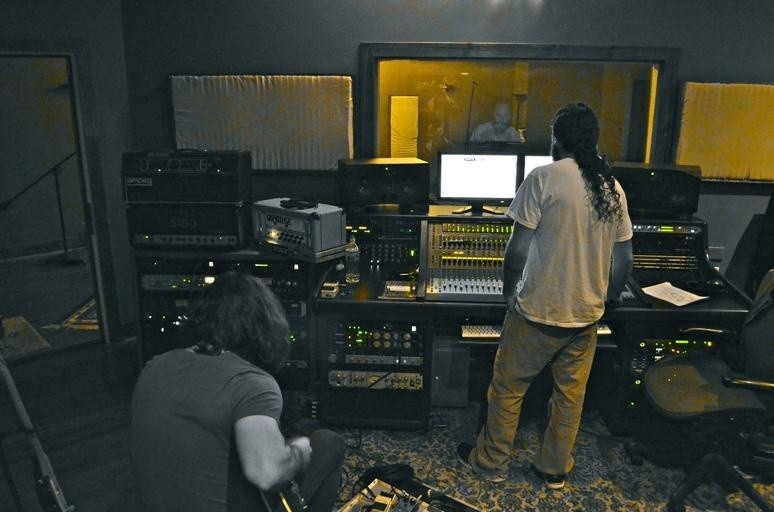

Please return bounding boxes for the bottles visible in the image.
[345,235,361,284]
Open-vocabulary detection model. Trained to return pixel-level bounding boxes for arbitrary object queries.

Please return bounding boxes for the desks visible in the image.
[305,261,755,436]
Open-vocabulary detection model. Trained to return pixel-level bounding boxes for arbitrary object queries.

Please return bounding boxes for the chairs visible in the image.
[640,267,774,512]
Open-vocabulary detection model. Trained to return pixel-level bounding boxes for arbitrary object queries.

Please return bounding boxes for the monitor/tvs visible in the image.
[521,151,555,180]
[437,150,521,215]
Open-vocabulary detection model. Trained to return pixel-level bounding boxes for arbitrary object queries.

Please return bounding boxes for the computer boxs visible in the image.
[431,334,470,409]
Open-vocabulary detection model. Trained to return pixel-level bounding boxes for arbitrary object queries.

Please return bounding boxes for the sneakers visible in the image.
[456,441,508,482]
[530,459,565,489]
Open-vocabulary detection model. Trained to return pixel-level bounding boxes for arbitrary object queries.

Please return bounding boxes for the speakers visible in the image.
[610,160,702,218]
[338,156,430,214]
[121,146,254,250]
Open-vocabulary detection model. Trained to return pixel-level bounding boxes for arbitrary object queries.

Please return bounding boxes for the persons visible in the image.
[122,269,348,511]
[465,100,522,142]
[454,99,636,492]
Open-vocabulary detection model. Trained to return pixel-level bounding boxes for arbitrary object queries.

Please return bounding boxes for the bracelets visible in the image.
[290,443,305,475]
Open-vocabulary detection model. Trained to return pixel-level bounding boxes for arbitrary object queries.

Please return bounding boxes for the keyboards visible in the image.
[460,322,613,340]
